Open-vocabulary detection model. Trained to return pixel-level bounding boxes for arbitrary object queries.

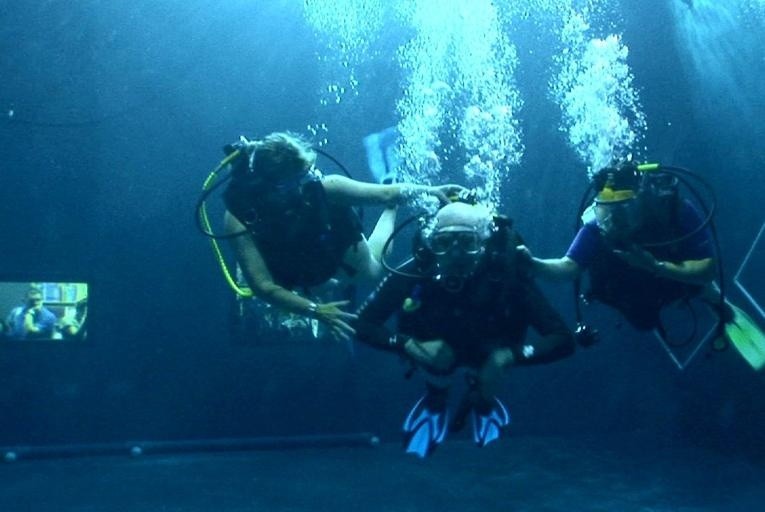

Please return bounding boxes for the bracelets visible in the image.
[307,303,317,317]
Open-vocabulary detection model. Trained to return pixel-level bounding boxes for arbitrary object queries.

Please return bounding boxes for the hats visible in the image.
[434,202,491,240]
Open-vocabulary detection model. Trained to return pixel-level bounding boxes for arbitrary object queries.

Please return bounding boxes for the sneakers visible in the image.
[712,301,733,323]
[464,380,494,414]
[424,384,447,412]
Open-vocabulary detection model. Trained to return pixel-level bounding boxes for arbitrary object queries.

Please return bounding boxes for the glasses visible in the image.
[592,200,642,230]
[427,225,484,256]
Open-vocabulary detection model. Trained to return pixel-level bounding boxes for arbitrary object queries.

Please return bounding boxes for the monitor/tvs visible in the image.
[0,281,88,342]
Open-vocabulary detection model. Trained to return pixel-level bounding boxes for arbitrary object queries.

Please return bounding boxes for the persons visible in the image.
[3,287,57,340]
[194,130,470,342]
[515,158,765,372]
[60,297,87,340]
[347,199,574,460]
